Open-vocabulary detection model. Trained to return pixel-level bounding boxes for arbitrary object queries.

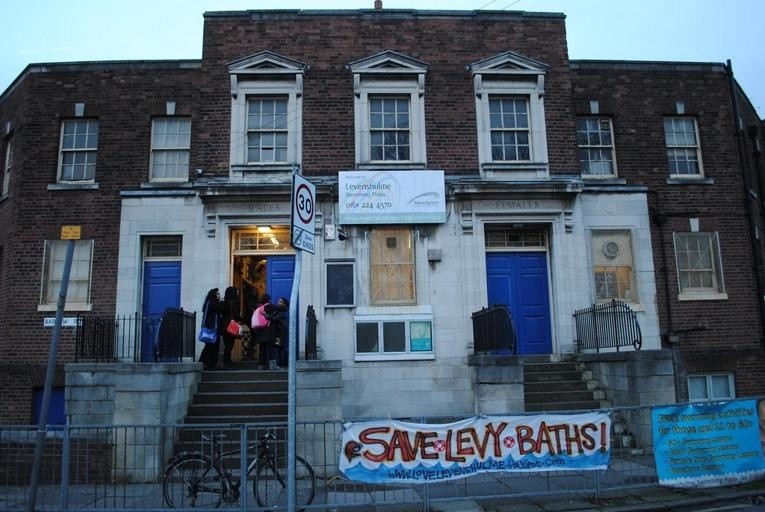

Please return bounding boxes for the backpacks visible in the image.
[251,302,271,330]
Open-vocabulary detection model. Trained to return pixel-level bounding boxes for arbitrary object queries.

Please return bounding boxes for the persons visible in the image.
[199,284,289,370]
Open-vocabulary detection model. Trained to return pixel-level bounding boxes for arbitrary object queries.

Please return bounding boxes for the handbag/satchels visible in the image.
[199,327,218,344]
[271,337,285,348]
[228,320,240,336]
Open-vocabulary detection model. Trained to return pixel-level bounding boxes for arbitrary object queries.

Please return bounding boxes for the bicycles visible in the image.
[162,429,320,512]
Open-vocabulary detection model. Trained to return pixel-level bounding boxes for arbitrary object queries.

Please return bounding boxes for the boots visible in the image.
[269,360,280,369]
[258,365,268,370]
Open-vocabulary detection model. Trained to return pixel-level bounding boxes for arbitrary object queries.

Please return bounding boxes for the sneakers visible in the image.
[224,367,235,370]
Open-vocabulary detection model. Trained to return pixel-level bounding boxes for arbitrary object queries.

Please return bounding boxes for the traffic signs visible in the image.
[296,183,315,226]
